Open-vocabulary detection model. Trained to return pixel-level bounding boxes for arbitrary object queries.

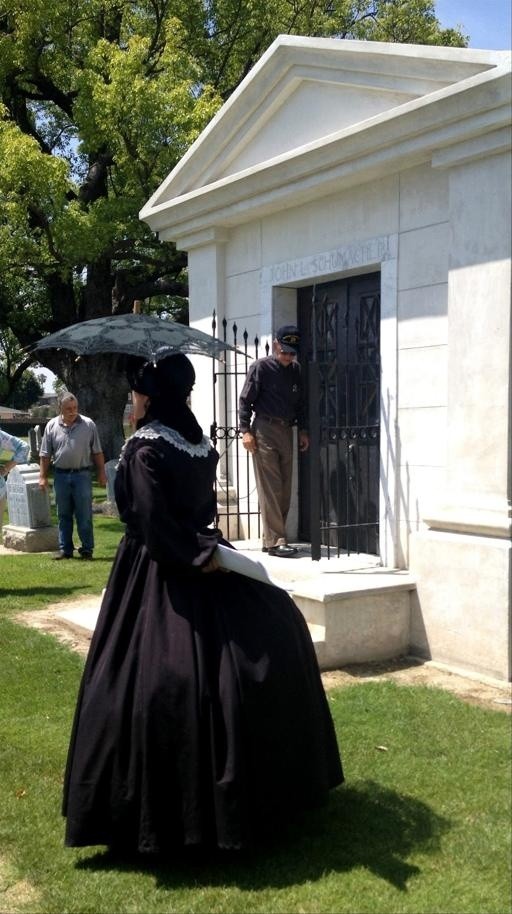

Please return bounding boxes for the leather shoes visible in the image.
[262,545,297,556]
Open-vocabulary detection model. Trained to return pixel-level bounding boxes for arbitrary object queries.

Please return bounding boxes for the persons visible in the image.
[38,392,109,560]
[239,325,310,557]
[64,351,345,852]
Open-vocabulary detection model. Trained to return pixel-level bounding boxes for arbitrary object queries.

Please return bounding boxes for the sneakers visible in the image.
[81,552,93,561]
[54,552,73,559]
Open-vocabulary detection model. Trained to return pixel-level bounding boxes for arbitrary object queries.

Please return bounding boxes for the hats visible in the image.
[128,349,196,398]
[276,325,301,353]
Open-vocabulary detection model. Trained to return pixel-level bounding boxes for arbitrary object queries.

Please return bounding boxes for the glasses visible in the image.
[280,345,296,356]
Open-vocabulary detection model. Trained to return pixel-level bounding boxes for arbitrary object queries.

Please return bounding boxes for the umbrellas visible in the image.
[25,300,252,368]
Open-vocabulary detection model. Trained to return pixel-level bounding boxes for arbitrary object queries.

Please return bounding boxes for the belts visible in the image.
[264,416,294,429]
[56,468,85,472]
